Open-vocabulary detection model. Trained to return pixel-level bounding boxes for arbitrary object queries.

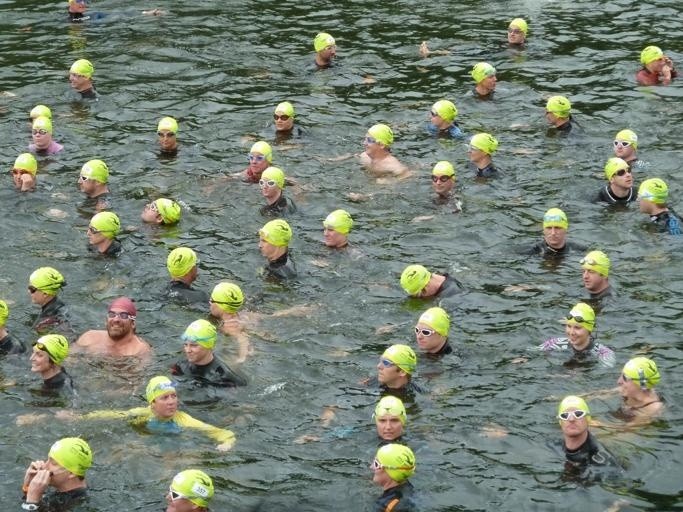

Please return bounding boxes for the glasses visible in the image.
[621,372,644,382]
[566,313,591,324]
[247,154,266,161]
[580,256,608,268]
[555,410,590,421]
[468,145,480,152]
[544,108,553,115]
[27,286,38,294]
[376,408,405,416]
[484,67,496,77]
[565,311,594,326]
[637,195,653,199]
[31,129,46,138]
[507,26,524,34]
[414,326,437,337]
[431,174,455,183]
[208,298,229,304]
[543,215,566,222]
[167,485,196,501]
[379,356,408,367]
[372,457,398,472]
[150,202,157,211]
[613,167,632,176]
[657,56,666,62]
[613,140,634,148]
[80,176,93,181]
[259,179,278,188]
[364,134,378,144]
[153,382,181,391]
[325,45,337,51]
[429,109,438,118]
[12,170,30,176]
[259,229,275,241]
[579,258,605,266]
[157,131,175,137]
[180,334,209,344]
[31,340,56,361]
[272,114,291,121]
[88,227,99,233]
[108,310,132,319]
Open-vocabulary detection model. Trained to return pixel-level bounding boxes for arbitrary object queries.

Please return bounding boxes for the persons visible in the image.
[140,197,181,227]
[467,132,498,179]
[368,443,421,512]
[28,333,75,399]
[273,100,296,132]
[0,299,25,357]
[26,116,64,157]
[371,395,407,446]
[421,99,466,141]
[399,264,465,299]
[541,303,616,371]
[415,306,454,359]
[258,219,298,280]
[464,62,497,97]
[209,281,303,363]
[69,59,100,98]
[65,296,150,359]
[636,177,681,235]
[20,437,92,512]
[257,166,297,218]
[580,250,615,300]
[85,211,122,260]
[545,96,573,131]
[77,157,114,211]
[507,18,528,44]
[68,0,93,21]
[240,139,272,183]
[430,160,456,197]
[165,468,214,512]
[592,157,640,204]
[546,394,621,474]
[28,266,72,329]
[305,32,342,74]
[25,104,52,127]
[618,356,663,419]
[638,45,679,86]
[53,375,236,452]
[613,129,639,170]
[12,153,37,193]
[156,116,178,150]
[170,319,244,388]
[359,124,401,172]
[162,246,207,306]
[322,209,353,249]
[375,343,417,403]
[529,207,585,256]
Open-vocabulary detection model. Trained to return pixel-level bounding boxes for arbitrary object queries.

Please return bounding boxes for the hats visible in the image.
[30,105,52,119]
[31,117,53,136]
[382,344,416,377]
[89,212,120,239]
[604,157,630,181]
[80,160,109,184]
[373,396,407,425]
[376,443,415,483]
[156,197,181,225]
[107,296,137,317]
[368,123,394,149]
[30,266,65,297]
[144,375,175,405]
[431,161,455,181]
[432,100,458,123]
[171,470,215,508]
[69,58,94,78]
[565,302,596,333]
[180,319,217,350]
[157,117,176,135]
[622,356,661,390]
[580,249,610,277]
[14,152,38,177]
[249,141,272,164]
[638,178,669,204]
[614,129,637,151]
[261,167,285,190]
[545,95,571,118]
[399,264,430,298]
[258,219,292,248]
[470,133,498,156]
[640,46,663,65]
[211,282,244,314]
[558,396,591,423]
[1,300,8,326]
[274,100,295,117]
[417,306,451,338]
[543,208,569,231]
[37,333,68,366]
[166,247,196,280]
[313,33,335,52]
[509,17,528,35]
[322,210,353,235]
[49,437,93,477]
[471,62,495,84]
